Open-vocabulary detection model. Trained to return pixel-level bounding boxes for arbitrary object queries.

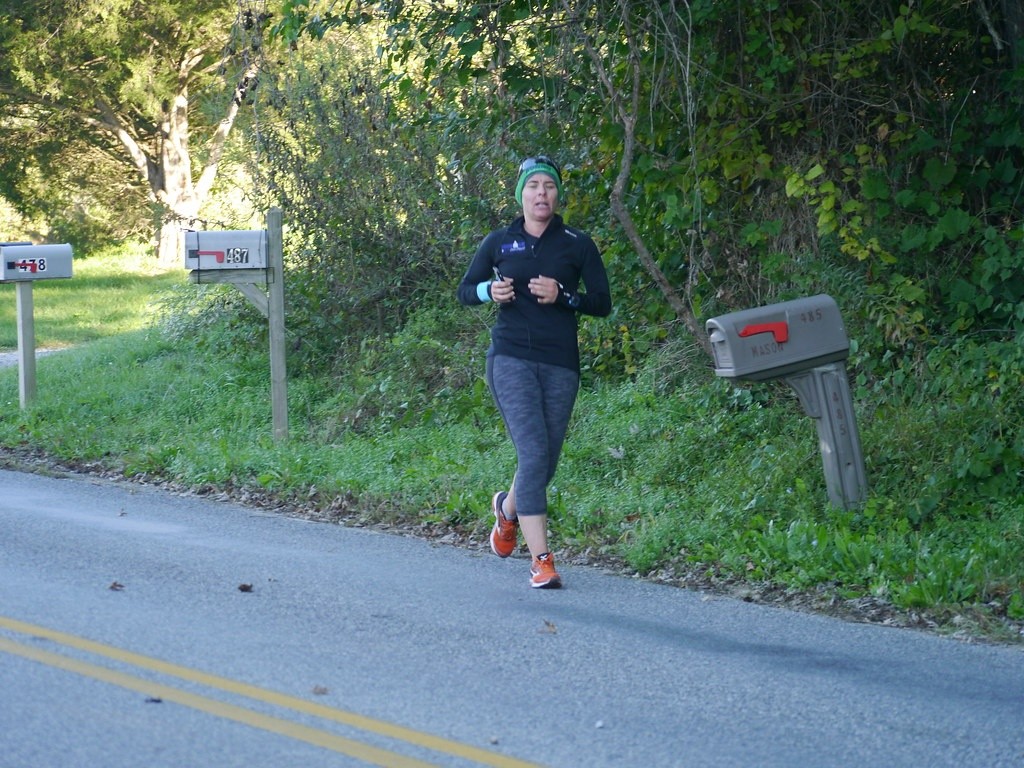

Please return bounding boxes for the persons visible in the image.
[457,156,612,588]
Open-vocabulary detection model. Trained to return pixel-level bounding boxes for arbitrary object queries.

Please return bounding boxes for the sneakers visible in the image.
[529,552,561,587]
[491,491,518,557]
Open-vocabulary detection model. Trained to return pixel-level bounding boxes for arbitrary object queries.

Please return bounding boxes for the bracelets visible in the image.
[477,280,493,302]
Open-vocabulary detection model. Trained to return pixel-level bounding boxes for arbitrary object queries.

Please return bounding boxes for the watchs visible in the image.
[569,288,581,311]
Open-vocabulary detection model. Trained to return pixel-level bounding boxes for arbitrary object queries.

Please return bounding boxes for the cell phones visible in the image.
[493,266,514,302]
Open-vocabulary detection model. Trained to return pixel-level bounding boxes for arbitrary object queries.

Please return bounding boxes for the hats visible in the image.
[514,155,562,206]
